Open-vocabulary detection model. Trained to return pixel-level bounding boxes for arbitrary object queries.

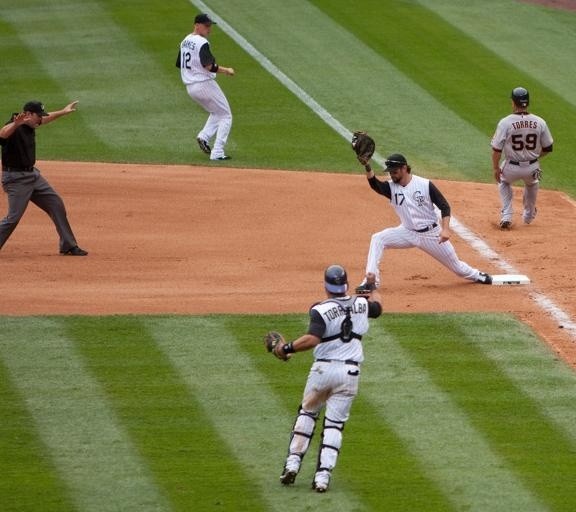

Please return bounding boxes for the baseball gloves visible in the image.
[263,331,291,361]
[351,130,375,165]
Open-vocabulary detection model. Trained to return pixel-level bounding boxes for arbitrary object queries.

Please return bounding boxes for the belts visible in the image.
[315,357,360,367]
[2,165,33,173]
[403,221,439,234]
[508,158,538,166]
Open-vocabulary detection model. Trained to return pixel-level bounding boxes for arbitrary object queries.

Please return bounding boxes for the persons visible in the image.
[491,85,554,228]
[353,130,492,294]
[266,264,383,491]
[1,99,89,256]
[175,14,236,162]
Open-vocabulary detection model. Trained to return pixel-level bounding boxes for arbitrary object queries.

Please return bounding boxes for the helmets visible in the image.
[509,86,530,107]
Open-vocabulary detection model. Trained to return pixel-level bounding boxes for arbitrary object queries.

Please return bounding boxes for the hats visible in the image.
[22,100,50,117]
[194,13,218,26]
[382,153,408,173]
[323,263,350,295]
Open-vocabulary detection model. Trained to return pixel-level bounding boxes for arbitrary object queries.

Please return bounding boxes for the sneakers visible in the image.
[312,480,329,492]
[57,244,89,257]
[209,155,232,161]
[196,137,212,155]
[478,272,493,285]
[523,207,538,225]
[279,466,298,486]
[498,220,513,229]
[353,271,381,293]
